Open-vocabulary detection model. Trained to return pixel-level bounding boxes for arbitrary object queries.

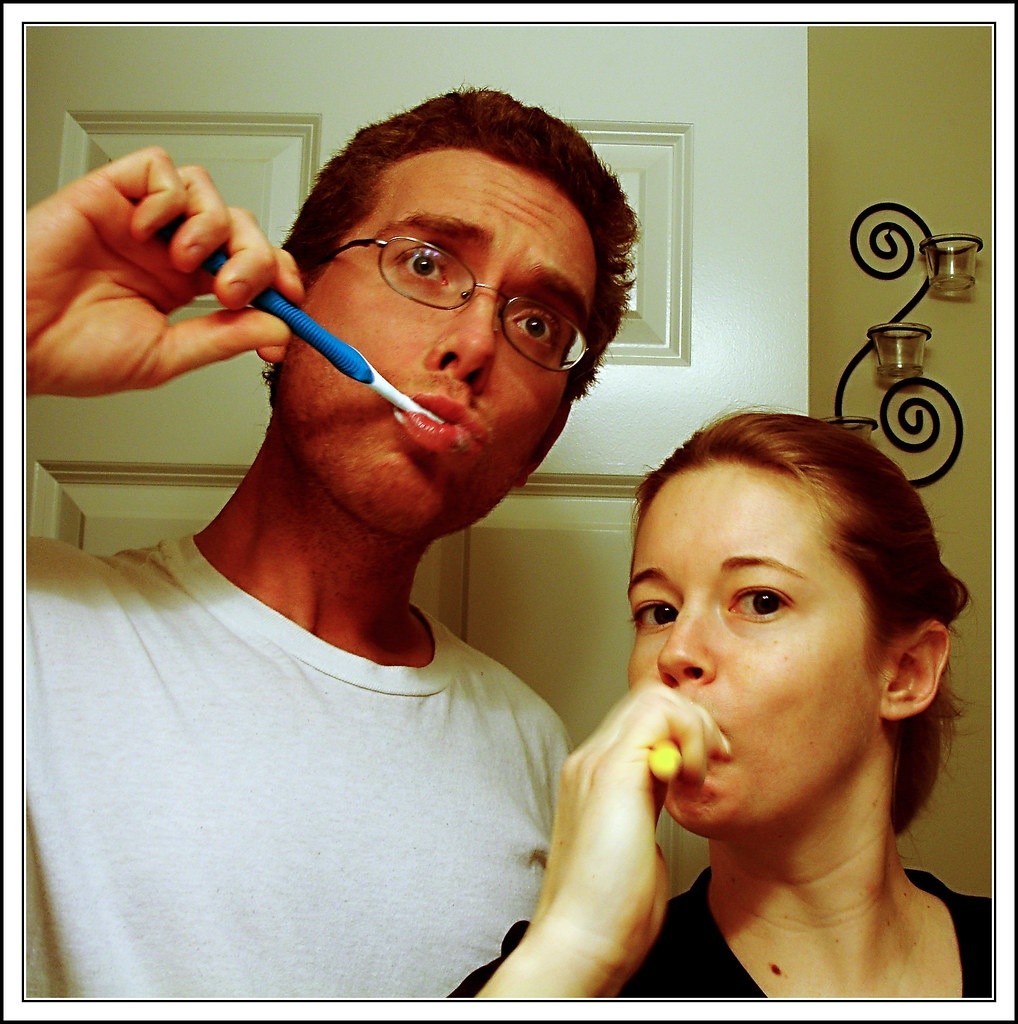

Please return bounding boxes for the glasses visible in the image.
[316,236,590,373]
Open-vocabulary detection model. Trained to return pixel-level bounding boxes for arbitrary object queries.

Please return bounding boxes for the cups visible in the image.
[868,322,932,378]
[817,416,873,441]
[919,233,983,290]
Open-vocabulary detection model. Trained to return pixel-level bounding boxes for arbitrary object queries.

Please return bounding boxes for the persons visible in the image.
[447,412,992,999]
[23,86,640,998]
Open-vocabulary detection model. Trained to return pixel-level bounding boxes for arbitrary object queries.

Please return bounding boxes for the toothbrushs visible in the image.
[646,733,685,786]
[153,211,447,429]
